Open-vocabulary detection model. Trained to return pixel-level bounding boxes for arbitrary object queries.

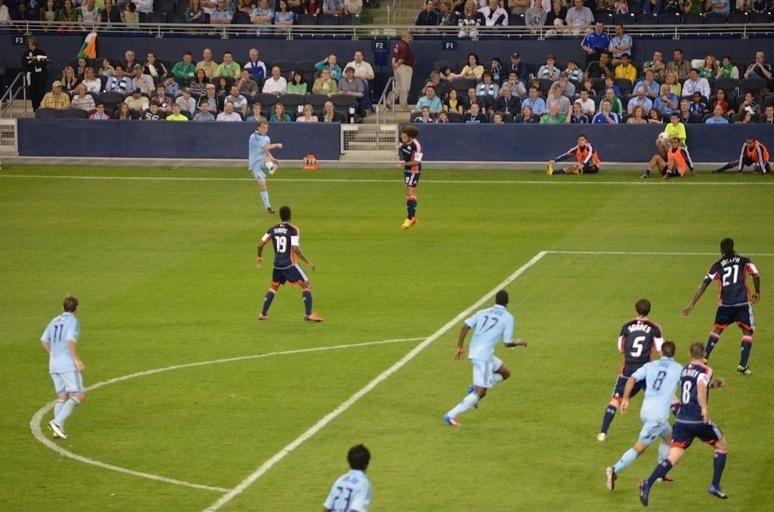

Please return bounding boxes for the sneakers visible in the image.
[606,466,617,490]
[443,414,459,426]
[640,475,673,506]
[304,314,323,321]
[468,385,479,409]
[48,420,68,440]
[709,485,728,499]
[400,216,416,230]
[737,364,752,375]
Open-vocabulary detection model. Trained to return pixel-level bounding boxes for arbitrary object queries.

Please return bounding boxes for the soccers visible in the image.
[264,160,273,170]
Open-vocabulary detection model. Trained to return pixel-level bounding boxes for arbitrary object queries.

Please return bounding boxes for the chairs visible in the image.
[595,10,774,37]
[416,58,773,124]
[507,14,528,39]
[31,55,370,124]
[140,0,357,39]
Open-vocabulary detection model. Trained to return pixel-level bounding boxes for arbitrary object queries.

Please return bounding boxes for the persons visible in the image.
[1,1,774,126]
[641,137,695,179]
[442,291,527,425]
[397,126,423,228]
[656,113,687,161]
[40,296,86,438]
[597,300,681,441]
[711,137,771,175]
[249,120,283,214]
[605,342,684,492]
[639,340,730,505]
[684,239,761,376]
[256,206,323,322]
[548,134,600,174]
[324,443,371,511]
